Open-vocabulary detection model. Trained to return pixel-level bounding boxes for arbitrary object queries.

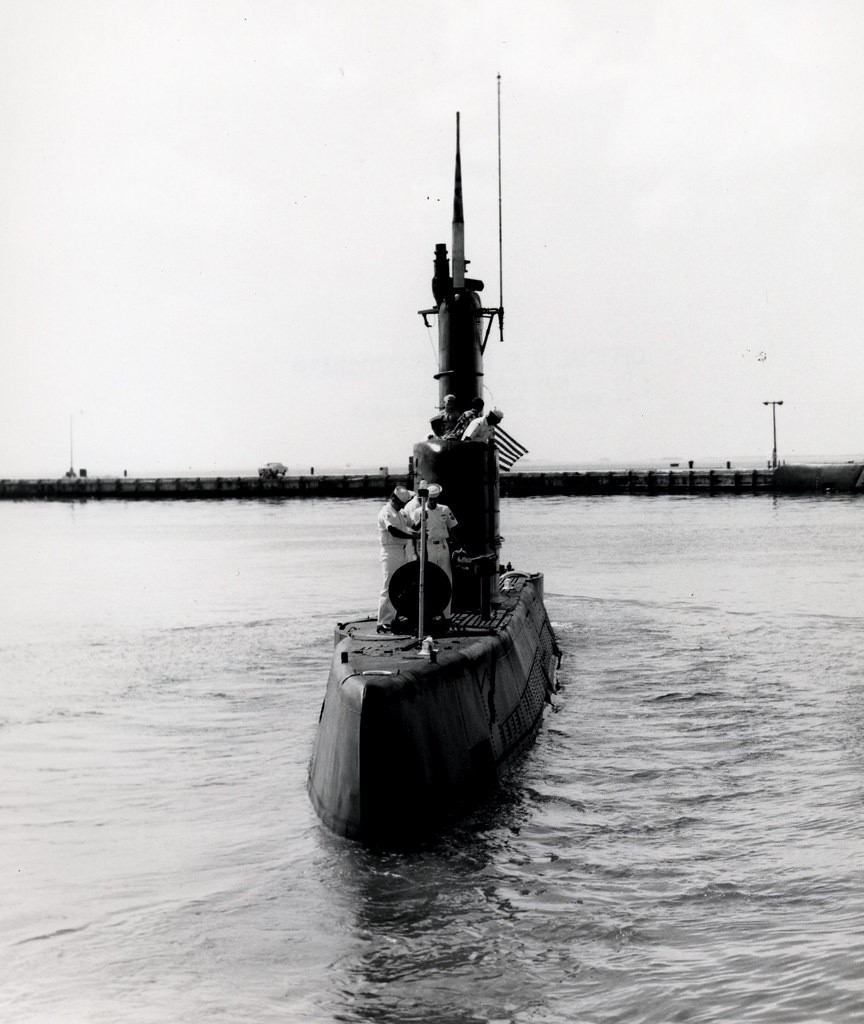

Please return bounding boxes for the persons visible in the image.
[438,394,460,431]
[377,482,458,628]
[461,409,503,444]
[452,398,484,436]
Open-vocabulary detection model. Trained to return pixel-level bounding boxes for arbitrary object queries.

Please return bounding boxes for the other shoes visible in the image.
[377,624,392,634]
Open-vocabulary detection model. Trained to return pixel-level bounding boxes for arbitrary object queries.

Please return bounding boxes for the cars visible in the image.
[258,462,288,477]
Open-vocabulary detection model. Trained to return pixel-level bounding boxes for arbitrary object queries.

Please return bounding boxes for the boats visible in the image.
[303,72,564,854]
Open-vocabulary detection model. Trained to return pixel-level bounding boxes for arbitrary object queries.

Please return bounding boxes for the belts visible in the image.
[403,545,406,549]
[433,540,443,544]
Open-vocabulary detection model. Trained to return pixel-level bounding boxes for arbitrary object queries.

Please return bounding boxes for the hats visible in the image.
[408,489,417,497]
[426,483,442,498]
[491,406,503,418]
[418,480,427,489]
[394,485,411,502]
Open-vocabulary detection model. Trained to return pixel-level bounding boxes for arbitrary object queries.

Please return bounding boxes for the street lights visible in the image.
[762,400,784,467]
[69,409,83,478]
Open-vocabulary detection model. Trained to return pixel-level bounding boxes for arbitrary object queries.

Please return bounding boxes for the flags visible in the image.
[492,424,527,472]
